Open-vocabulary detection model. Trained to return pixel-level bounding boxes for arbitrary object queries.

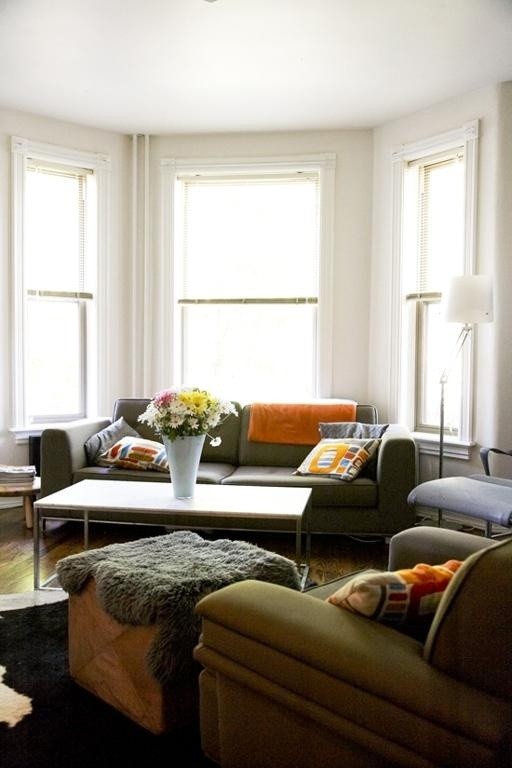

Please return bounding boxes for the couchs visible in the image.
[40,397,417,535]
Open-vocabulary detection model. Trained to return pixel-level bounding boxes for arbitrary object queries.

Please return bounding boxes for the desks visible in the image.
[0,476,42,529]
[32,478,312,595]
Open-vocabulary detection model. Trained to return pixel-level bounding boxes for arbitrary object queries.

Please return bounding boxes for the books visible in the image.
[0,465,36,487]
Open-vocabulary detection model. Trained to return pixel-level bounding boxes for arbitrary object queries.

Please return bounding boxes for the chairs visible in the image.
[410,447,512,539]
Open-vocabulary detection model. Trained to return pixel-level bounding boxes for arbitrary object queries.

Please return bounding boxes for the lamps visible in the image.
[438,274,495,479]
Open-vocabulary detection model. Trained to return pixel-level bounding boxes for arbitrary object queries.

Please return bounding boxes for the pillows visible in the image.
[84,417,143,469]
[325,558,472,625]
[292,437,383,483]
[319,422,390,479]
[96,435,170,473]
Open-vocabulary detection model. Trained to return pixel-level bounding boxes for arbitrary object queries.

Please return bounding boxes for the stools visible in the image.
[55,531,304,741]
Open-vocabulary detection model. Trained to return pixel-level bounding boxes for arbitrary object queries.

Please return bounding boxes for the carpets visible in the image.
[1,599,200,767]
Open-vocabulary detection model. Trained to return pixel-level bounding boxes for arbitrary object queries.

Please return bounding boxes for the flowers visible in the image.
[137,387,239,448]
